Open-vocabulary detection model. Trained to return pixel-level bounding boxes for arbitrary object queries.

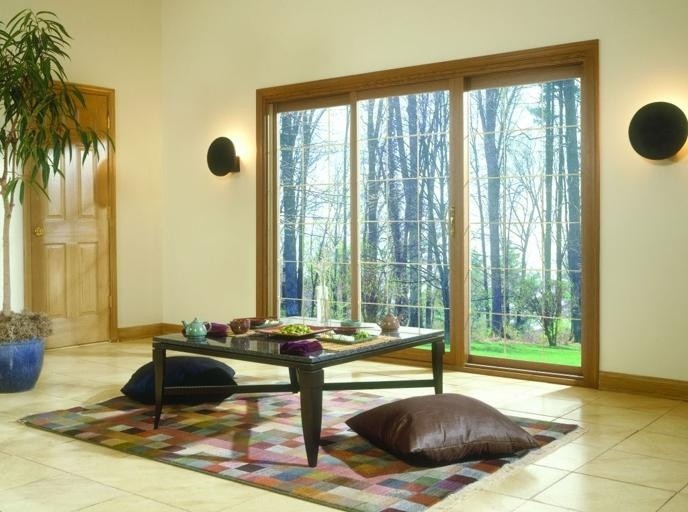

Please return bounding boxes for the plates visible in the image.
[255,324,333,338]
[315,332,378,345]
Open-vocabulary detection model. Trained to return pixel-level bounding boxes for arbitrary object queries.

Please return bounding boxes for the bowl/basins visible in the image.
[340,318,362,328]
[333,327,357,336]
[230,318,250,334]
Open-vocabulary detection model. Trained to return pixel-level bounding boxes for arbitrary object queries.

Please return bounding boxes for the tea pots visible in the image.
[376,310,405,333]
[181,317,212,341]
